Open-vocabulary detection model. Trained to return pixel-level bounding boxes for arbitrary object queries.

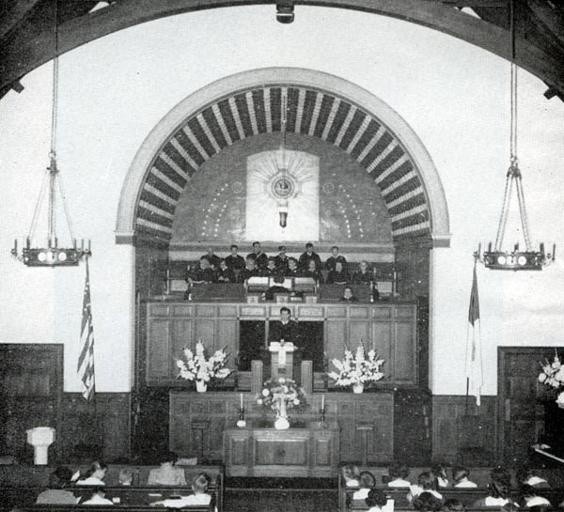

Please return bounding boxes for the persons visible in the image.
[237,253,264,284]
[71,459,108,488]
[350,258,374,284]
[245,241,267,264]
[297,242,321,266]
[274,245,289,264]
[210,258,236,285]
[223,245,246,268]
[324,260,348,285]
[338,457,564,512]
[33,467,79,505]
[199,246,222,269]
[270,306,299,345]
[78,484,116,505]
[325,246,346,270]
[115,466,135,488]
[279,257,306,279]
[300,258,324,287]
[147,470,219,511]
[259,256,284,278]
[141,449,188,487]
[189,258,217,283]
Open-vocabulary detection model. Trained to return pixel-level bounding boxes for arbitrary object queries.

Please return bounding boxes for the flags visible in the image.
[461,265,485,406]
[74,270,98,403]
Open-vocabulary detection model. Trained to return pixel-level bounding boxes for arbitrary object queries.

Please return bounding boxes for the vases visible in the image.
[353,382,364,393]
[270,408,296,430]
[194,378,208,392]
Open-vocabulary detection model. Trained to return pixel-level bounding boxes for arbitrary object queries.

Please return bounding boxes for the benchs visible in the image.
[0,462,225,511]
[188,270,373,302]
[337,466,563,511]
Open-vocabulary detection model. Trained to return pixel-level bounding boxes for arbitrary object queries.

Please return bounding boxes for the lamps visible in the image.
[10,0,94,268]
[473,1,560,271]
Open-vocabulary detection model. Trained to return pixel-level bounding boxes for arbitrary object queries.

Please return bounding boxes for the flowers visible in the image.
[537,348,564,409]
[254,376,310,417]
[173,340,235,382]
[322,338,391,386]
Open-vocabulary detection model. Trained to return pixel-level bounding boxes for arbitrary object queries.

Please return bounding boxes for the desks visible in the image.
[223,427,339,477]
[146,303,420,388]
